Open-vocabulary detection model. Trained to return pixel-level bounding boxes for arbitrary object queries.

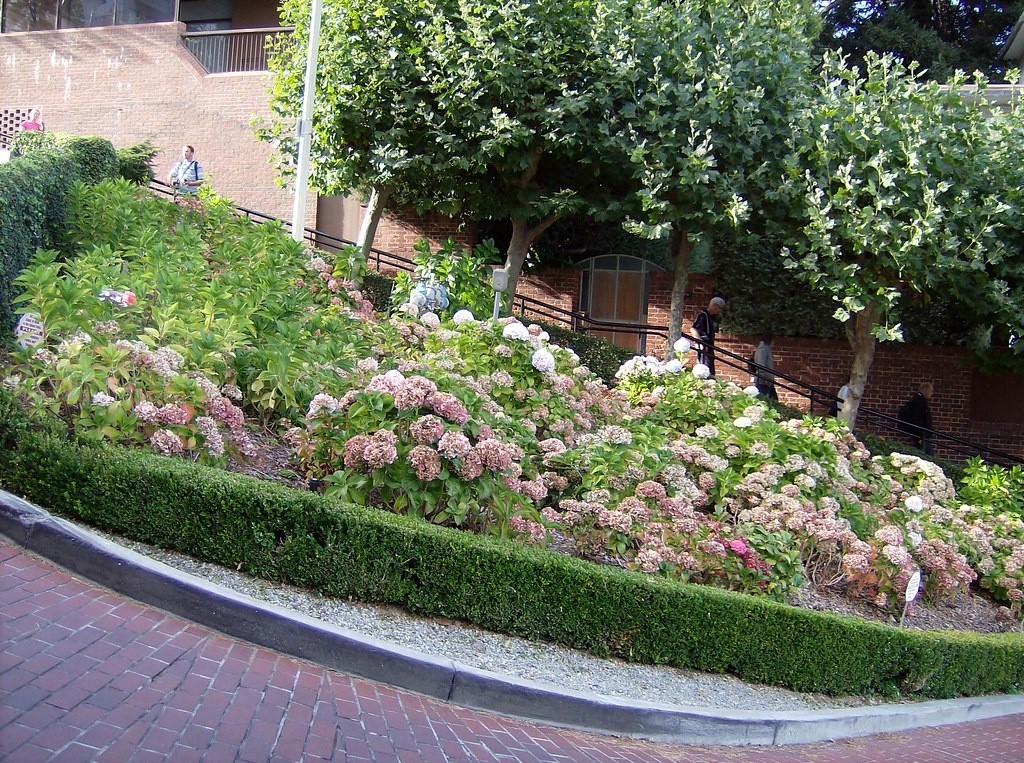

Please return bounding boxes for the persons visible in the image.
[895,382,934,455]
[754,333,778,402]
[167,145,203,204]
[690,297,726,376]
[19,109,45,133]
[827,379,851,418]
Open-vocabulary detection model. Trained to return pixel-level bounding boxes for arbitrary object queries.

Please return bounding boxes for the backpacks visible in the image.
[748,352,758,374]
[897,397,914,434]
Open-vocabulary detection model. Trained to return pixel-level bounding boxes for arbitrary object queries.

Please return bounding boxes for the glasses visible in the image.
[716,304,723,310]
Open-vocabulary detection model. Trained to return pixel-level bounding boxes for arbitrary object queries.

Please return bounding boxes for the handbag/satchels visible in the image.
[828,399,837,418]
